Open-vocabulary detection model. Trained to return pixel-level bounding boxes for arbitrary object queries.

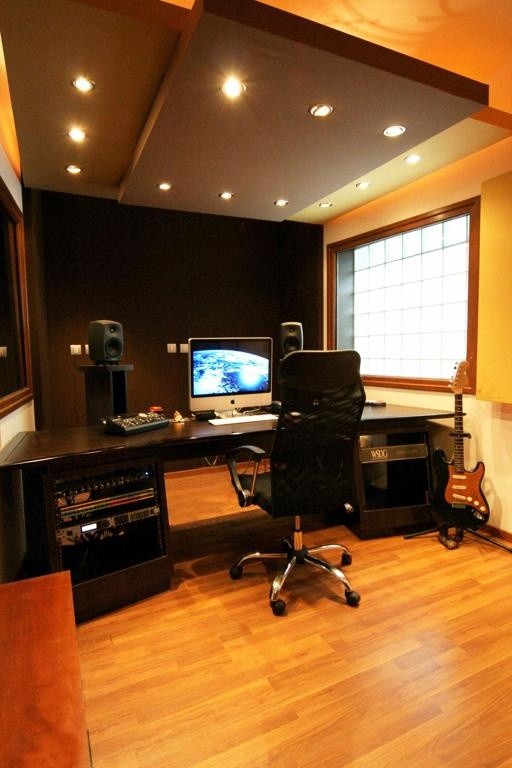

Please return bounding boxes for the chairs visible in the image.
[227,348,371,618]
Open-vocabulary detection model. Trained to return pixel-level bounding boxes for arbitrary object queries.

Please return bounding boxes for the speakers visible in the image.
[279,322,304,359]
[88,319,124,365]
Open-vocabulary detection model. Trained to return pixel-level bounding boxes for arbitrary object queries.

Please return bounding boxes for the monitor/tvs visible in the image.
[188,336,273,418]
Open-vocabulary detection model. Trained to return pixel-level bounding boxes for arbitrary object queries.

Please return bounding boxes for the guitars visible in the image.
[429,361,489,530]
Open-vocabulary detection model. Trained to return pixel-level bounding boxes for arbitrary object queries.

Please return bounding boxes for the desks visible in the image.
[0,395,483,626]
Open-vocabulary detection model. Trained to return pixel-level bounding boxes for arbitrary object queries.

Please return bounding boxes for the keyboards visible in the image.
[208,413,280,426]
